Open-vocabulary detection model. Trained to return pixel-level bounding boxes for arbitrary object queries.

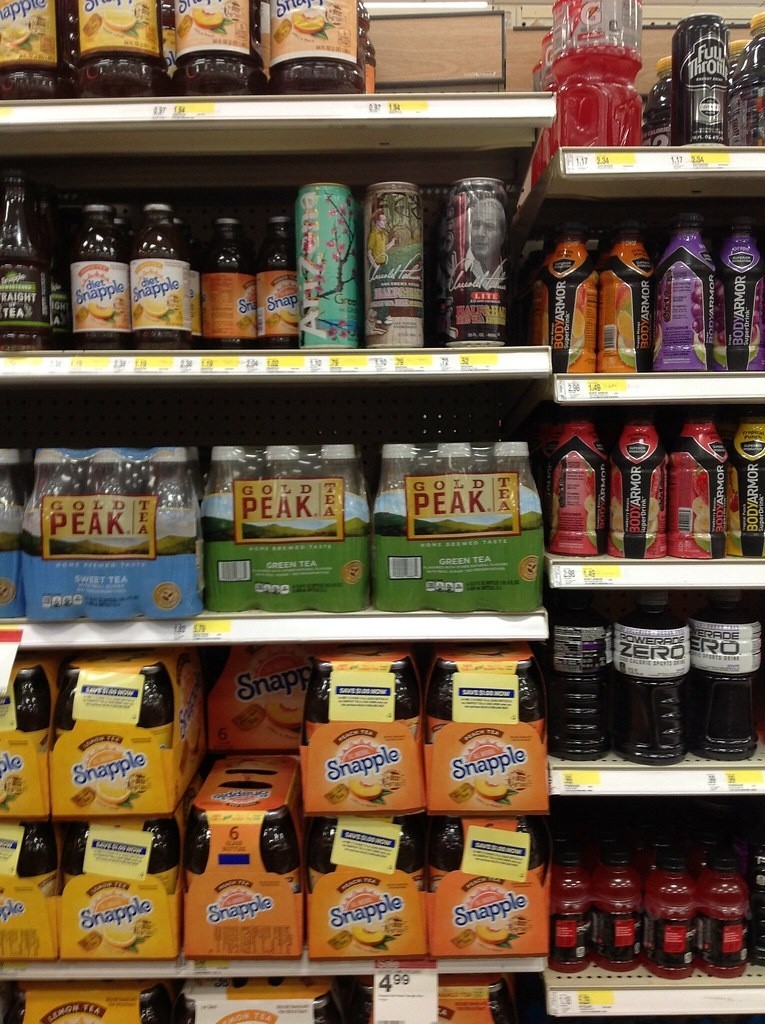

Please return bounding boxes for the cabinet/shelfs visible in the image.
[0,90,563,976]
[540,141,765,1019]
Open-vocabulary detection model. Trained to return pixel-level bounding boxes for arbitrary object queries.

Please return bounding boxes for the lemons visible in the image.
[102,929,137,948]
[102,11,137,31]
[97,784,130,803]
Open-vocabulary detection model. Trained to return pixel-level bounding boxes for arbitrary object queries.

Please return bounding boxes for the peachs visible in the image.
[145,303,169,318]
[348,778,509,945]
[88,302,115,319]
[192,7,224,29]
[292,12,325,34]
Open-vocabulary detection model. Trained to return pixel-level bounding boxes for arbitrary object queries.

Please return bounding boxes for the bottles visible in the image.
[544,590,765,766]
[0,176,300,351]
[727,13,765,146]
[0,0,377,100]
[0,442,545,621]
[547,830,765,980]
[540,408,765,559]
[523,211,765,373]
[641,55,672,147]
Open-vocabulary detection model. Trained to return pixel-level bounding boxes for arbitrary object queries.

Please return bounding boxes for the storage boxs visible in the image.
[0,528,554,1024]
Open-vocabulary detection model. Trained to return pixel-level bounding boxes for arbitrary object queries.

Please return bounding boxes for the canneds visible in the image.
[298,176,508,346]
[672,14,727,146]
